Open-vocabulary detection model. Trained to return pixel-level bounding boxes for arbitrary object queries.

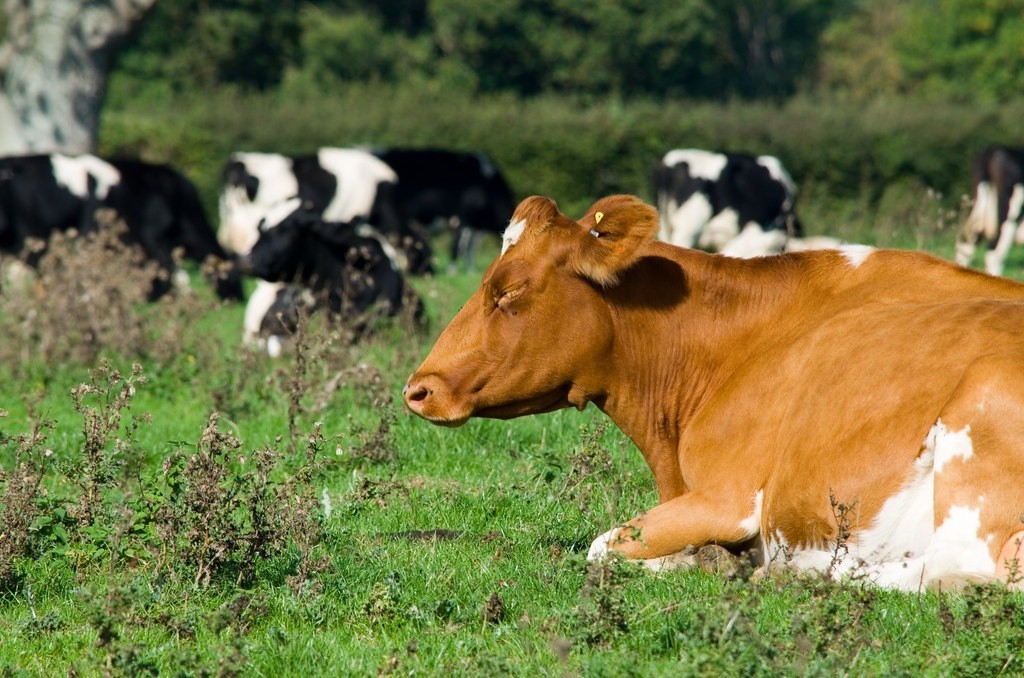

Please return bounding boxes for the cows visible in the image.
[403,194,1024,602]
[647,150,842,259]
[954,143,1024,278]
[0,145,517,356]
